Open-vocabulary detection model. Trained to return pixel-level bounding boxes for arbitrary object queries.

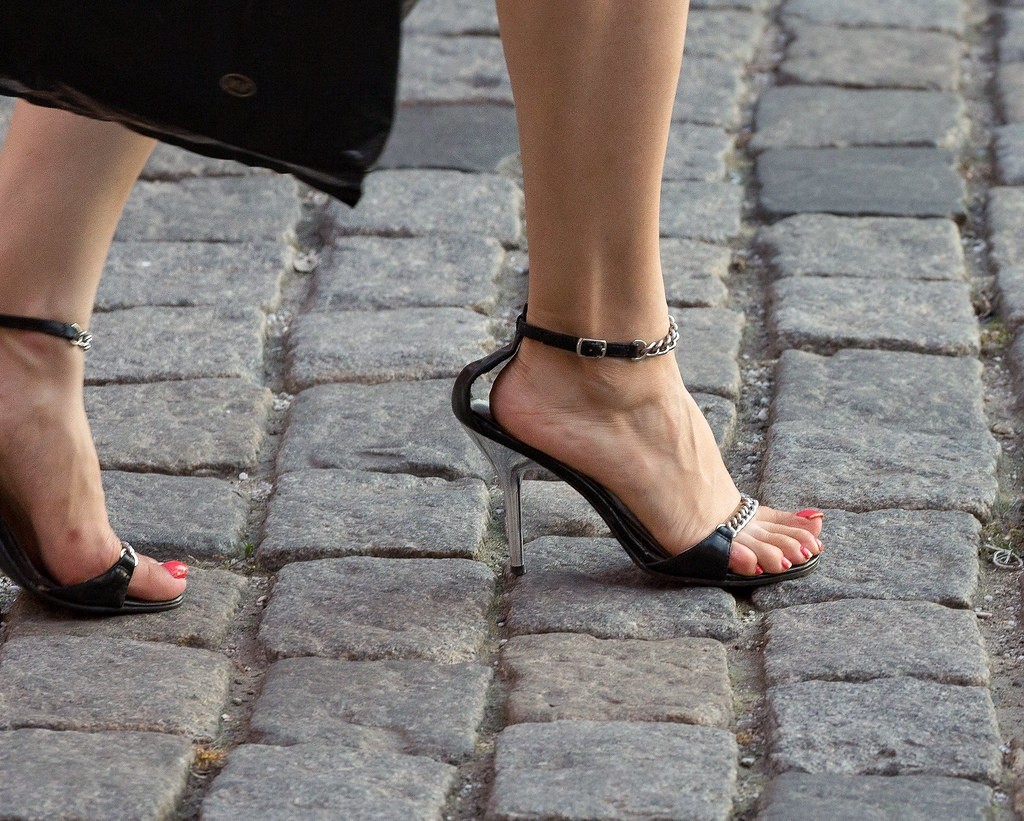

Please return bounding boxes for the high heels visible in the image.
[450,302,821,587]
[0,313,184,611]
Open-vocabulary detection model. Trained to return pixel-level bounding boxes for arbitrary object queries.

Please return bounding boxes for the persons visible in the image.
[0,0,824,618]
